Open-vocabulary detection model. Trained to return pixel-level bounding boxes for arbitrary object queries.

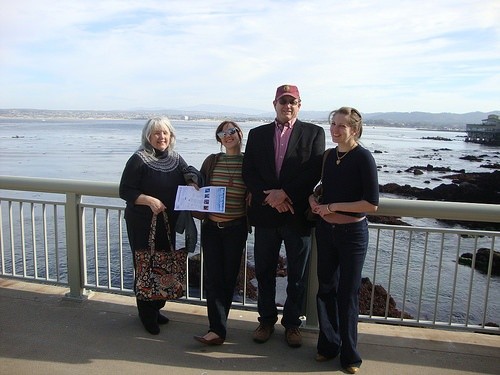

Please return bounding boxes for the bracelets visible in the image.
[326,203,336,214]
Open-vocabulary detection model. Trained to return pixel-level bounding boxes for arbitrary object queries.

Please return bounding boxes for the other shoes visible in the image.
[315,353,328,361]
[142,320,161,334]
[348,366,358,373]
[194,331,224,345]
[158,314,169,325]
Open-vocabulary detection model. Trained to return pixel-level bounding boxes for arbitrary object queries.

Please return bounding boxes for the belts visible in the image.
[206,218,245,229]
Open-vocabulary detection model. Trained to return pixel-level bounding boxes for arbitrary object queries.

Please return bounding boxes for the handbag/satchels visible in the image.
[135,210,188,301]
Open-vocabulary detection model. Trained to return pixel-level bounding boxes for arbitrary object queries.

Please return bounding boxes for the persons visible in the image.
[117,116,202,336]
[194,122,252,346]
[309,106,380,374]
[242,84,325,348]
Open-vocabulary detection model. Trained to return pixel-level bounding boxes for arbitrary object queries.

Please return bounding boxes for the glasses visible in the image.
[217,128,238,140]
[279,98,300,106]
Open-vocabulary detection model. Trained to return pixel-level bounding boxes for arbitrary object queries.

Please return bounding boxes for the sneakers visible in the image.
[286,327,302,345]
[254,323,274,343]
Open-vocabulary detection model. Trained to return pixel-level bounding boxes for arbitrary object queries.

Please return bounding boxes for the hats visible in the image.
[275,85,300,101]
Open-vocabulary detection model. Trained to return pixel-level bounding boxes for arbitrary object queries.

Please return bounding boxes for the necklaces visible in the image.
[335,142,357,165]
[228,177,233,186]
